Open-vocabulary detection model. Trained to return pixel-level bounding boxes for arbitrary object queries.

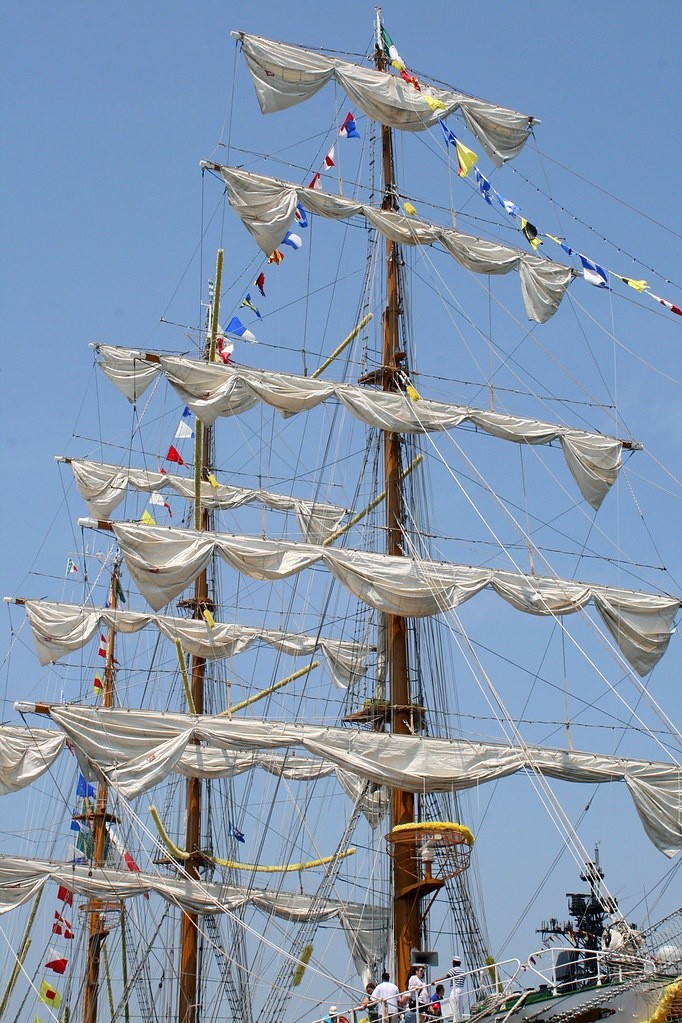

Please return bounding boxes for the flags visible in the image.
[474,168,682,315]
[67,560,78,575]
[216,231,303,364]
[92,579,127,695]
[35,774,99,1023]
[295,113,360,227]
[382,30,478,177]
[139,406,199,527]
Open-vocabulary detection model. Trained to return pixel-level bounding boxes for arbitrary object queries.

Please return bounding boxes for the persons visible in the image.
[322,1006,337,1023]
[359,956,466,1023]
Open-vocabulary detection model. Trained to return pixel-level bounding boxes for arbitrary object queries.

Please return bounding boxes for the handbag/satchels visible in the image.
[409,997,416,1012]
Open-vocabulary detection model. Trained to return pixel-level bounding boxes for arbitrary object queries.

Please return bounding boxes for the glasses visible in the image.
[419,969,424,973]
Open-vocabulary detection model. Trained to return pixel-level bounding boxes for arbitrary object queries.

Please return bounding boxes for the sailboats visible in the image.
[0,5,681,1022]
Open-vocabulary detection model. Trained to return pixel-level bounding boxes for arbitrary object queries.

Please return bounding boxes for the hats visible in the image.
[432,1003,440,1009]
[329,1006,338,1014]
[453,955,461,962]
